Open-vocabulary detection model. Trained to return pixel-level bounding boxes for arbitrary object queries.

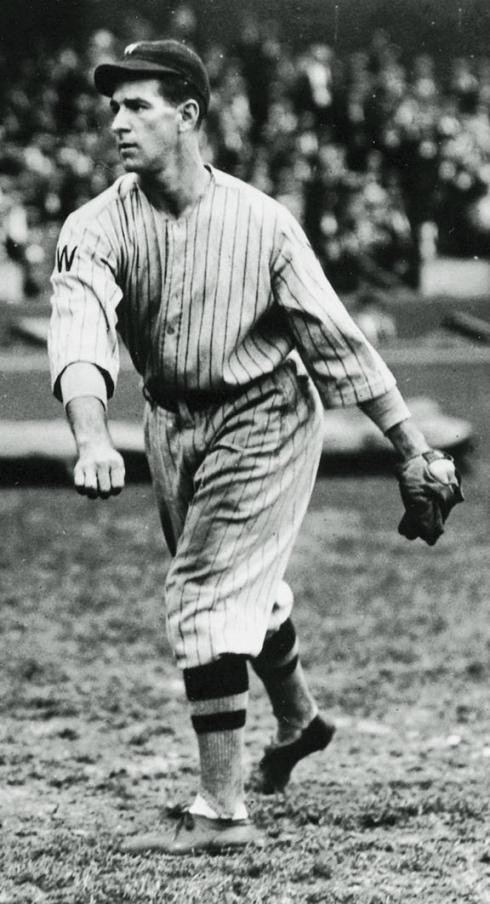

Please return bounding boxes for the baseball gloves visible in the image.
[397,445,464,544]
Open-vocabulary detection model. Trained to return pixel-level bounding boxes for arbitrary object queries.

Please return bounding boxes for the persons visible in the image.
[47,37,468,856]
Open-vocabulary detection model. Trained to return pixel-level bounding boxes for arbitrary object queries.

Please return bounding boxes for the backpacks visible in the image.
[95,41,210,105]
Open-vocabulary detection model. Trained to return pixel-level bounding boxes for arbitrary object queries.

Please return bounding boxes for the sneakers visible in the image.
[244,713,337,794]
[115,808,267,853]
